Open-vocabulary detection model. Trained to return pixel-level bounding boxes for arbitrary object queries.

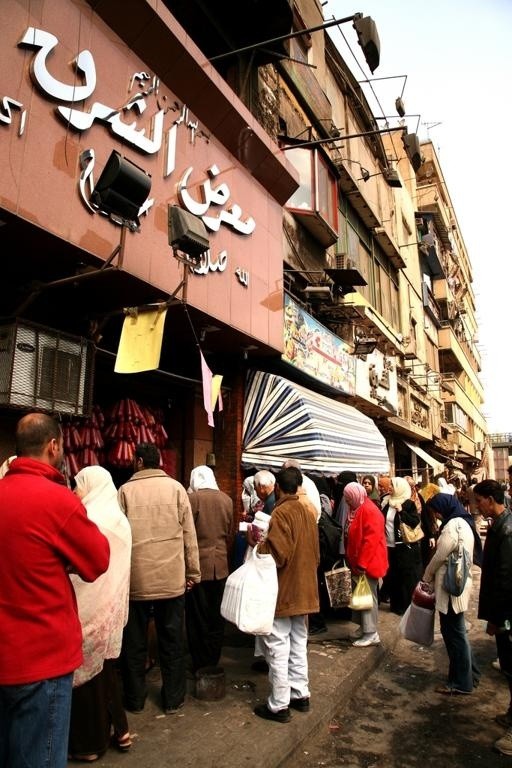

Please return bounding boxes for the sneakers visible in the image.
[496,713,510,727]
[350,626,380,647]
[434,684,455,692]
[494,727,512,754]
[254,704,290,723]
[289,697,309,712]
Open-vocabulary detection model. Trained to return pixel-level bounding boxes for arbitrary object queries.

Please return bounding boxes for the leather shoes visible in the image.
[133,709,145,714]
[308,625,328,635]
[165,703,183,713]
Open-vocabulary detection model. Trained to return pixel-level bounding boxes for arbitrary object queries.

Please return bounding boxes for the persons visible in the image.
[1,444,134,763]
[119,446,200,714]
[239,460,323,721]
[307,471,511,696]
[1,412,111,767]
[185,465,233,678]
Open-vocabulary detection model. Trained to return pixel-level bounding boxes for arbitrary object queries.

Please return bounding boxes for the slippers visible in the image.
[119,740,133,749]
[68,755,98,761]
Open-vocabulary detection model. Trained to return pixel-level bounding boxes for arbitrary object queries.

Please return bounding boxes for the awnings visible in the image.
[241,370,392,478]
[405,441,445,476]
[432,450,464,469]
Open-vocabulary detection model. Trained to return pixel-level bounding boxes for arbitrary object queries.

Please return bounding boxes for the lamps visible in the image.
[163,203,210,310]
[280,125,422,175]
[209,12,380,75]
[88,148,157,274]
[357,167,402,190]
[357,74,408,118]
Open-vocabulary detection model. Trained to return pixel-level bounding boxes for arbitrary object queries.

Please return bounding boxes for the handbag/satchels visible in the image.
[401,522,425,543]
[221,543,278,635]
[443,541,470,596]
[324,560,352,608]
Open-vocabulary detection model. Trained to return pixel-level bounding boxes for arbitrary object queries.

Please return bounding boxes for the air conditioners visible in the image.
[333,252,359,272]
[1,323,89,417]
